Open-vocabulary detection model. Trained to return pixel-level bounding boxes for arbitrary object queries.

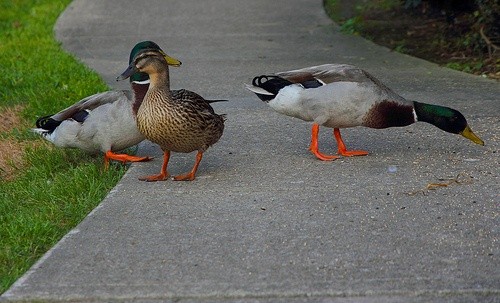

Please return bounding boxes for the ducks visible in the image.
[243,63,485,161]
[29,41,230,182]
